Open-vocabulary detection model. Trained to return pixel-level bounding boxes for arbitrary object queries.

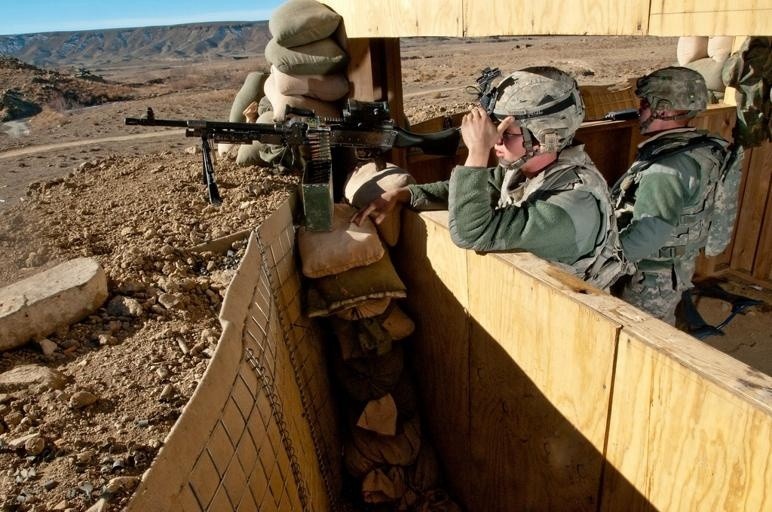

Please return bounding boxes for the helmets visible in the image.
[487,66,585,155]
[634,66,707,115]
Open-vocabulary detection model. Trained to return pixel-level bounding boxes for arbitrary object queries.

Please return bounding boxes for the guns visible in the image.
[125,98,463,232]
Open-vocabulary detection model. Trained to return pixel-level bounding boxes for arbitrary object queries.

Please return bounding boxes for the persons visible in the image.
[348,67,613,297]
[611,66,744,328]
